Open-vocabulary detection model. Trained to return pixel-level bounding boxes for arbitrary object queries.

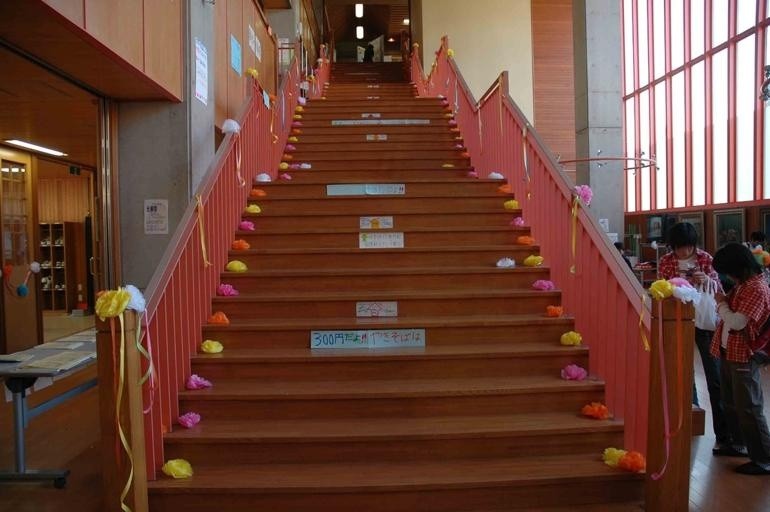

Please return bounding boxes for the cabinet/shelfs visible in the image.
[37,221,76,314]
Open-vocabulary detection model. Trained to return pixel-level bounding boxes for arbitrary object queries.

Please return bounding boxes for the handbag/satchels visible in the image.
[693,290,718,331]
[743,316,770,364]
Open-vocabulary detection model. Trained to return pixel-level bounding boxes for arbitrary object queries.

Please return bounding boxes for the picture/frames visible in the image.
[678,208,746,252]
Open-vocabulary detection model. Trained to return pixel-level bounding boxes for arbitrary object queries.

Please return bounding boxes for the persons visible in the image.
[613,242,631,268]
[655,220,723,442]
[363,44,374,62]
[706,242,770,476]
[742,230,764,268]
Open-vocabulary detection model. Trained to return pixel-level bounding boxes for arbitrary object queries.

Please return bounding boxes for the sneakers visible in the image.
[712,434,770,475]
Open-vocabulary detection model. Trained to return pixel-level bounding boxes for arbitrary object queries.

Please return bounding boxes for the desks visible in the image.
[0,327,99,491]
